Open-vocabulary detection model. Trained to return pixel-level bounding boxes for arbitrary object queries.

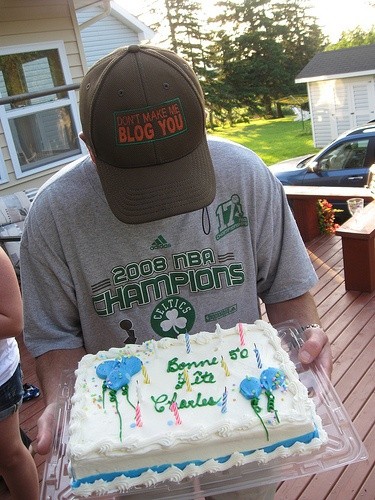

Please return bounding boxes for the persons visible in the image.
[20,45,332,500]
[0,244,39,500]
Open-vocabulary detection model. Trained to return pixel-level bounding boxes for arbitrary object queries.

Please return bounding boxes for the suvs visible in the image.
[263,122,375,224]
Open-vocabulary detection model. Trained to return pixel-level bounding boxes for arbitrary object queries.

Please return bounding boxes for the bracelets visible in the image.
[289,324,325,351]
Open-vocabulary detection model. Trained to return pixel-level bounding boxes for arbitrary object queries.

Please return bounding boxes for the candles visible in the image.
[140,361,150,384]
[153,338,160,359]
[253,342,262,369]
[136,380,142,401]
[221,386,228,414]
[135,401,143,427]
[220,356,230,376]
[185,329,190,353]
[238,319,245,346]
[184,367,192,392]
[170,401,182,425]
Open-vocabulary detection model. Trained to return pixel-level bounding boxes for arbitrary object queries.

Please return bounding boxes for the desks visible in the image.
[0,220,26,242]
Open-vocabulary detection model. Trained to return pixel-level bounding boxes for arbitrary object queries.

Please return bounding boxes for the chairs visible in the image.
[0,188,38,286]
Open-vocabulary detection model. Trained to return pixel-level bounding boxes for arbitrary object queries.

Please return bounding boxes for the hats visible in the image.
[79,44,216,224]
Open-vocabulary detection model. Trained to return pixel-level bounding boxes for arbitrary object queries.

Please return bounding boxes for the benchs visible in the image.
[283,185,375,292]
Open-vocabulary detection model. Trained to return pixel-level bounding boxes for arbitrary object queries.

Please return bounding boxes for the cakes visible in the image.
[65,319,329,498]
